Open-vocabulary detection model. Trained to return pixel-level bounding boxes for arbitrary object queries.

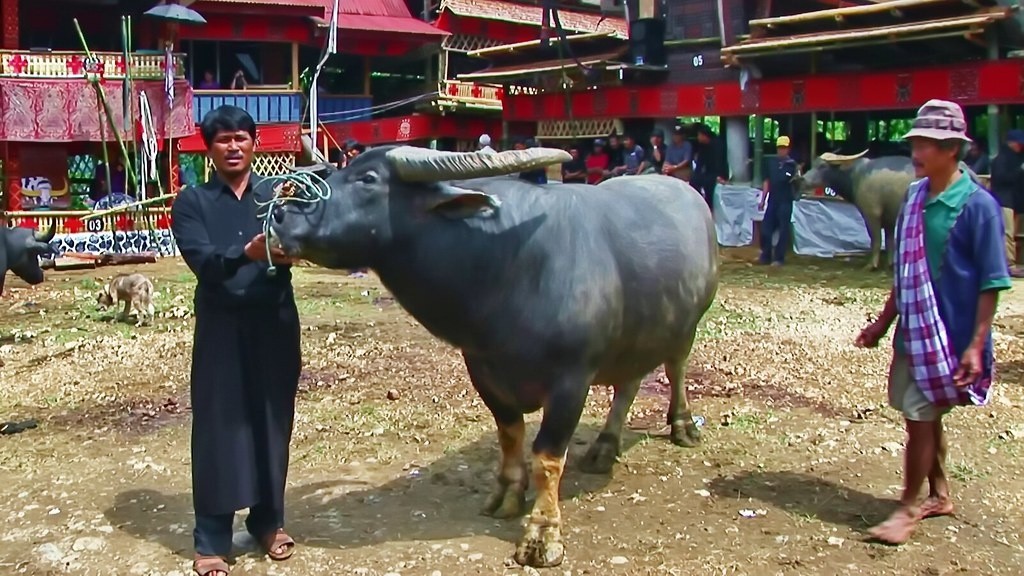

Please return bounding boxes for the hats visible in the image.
[1007,129,1024,144]
[776,136,790,146]
[593,139,605,146]
[479,134,491,145]
[648,129,664,137]
[901,99,973,142]
[672,124,684,134]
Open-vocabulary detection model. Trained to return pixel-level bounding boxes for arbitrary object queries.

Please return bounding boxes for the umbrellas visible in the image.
[144,3,208,49]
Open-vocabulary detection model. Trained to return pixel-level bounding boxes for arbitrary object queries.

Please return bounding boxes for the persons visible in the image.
[989,130,1024,278]
[231,71,248,90]
[753,135,797,266]
[168,104,312,576]
[854,97,1016,547]
[199,68,219,89]
[474,133,498,154]
[511,124,733,215]
[343,139,366,159]
[964,141,992,174]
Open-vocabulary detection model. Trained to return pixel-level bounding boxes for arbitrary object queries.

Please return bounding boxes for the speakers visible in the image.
[630,18,667,66]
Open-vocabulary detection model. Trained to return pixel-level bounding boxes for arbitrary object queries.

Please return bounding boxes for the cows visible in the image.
[269,142,719,565]
[802,146,987,272]
[0,216,57,298]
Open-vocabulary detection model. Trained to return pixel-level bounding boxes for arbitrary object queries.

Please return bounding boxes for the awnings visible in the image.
[333,13,453,38]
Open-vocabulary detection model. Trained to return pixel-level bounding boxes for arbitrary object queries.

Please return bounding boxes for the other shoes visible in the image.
[746,260,771,268]
[771,260,784,267]
[1009,269,1024,277]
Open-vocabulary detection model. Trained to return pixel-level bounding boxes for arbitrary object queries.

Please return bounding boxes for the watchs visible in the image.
[674,163,680,171]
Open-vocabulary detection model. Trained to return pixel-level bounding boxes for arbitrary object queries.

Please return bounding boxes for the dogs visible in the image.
[95,274,155,326]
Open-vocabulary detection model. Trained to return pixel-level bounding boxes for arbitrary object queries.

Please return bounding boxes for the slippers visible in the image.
[256,528,295,560]
[193,551,228,576]
